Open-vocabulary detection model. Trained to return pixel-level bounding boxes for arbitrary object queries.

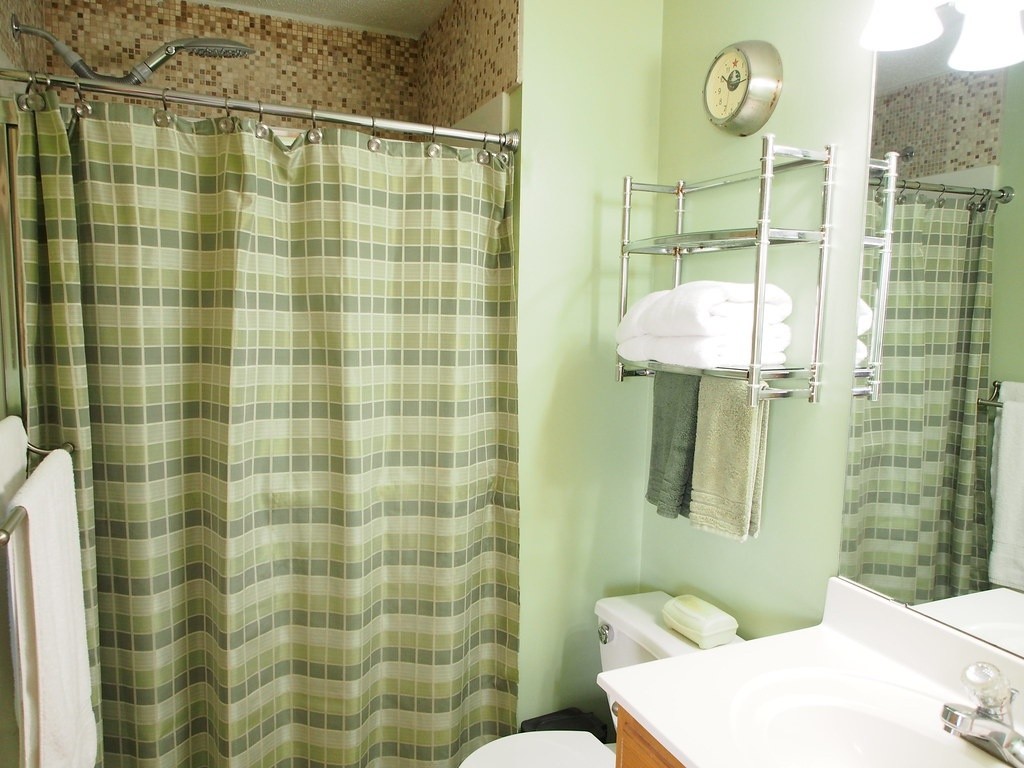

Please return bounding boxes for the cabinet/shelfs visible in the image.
[849,151,899,403]
[614,132,838,409]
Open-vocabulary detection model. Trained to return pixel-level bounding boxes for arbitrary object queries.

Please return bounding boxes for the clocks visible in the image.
[701,39,783,137]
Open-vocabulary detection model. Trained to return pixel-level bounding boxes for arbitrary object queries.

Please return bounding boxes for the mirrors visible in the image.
[835,0,1024,660]
